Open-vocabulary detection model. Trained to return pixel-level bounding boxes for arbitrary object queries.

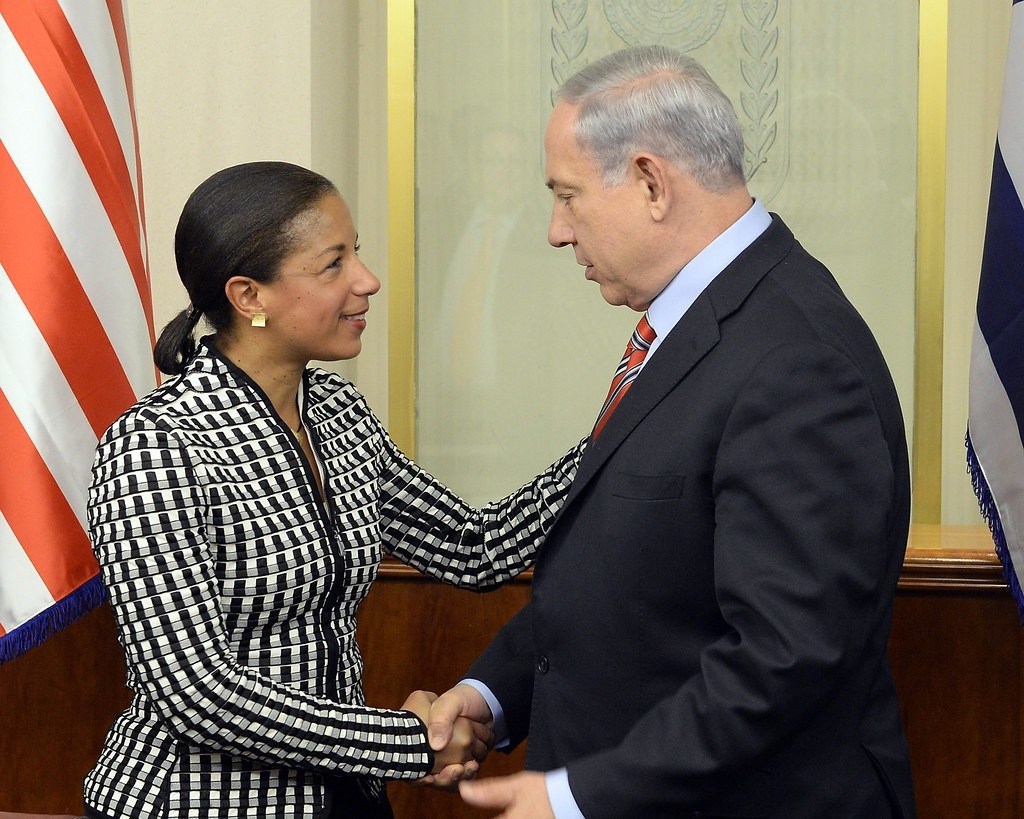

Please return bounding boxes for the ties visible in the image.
[592,313,657,446]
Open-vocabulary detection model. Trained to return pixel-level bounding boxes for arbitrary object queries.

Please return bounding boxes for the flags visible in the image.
[0,0,164,663]
[965,0,1024,619]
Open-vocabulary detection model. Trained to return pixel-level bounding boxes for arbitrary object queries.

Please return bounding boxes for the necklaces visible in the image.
[291,420,304,448]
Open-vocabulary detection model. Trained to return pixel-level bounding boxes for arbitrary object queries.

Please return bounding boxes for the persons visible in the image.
[428,46,916,819]
[82,162,590,819]
